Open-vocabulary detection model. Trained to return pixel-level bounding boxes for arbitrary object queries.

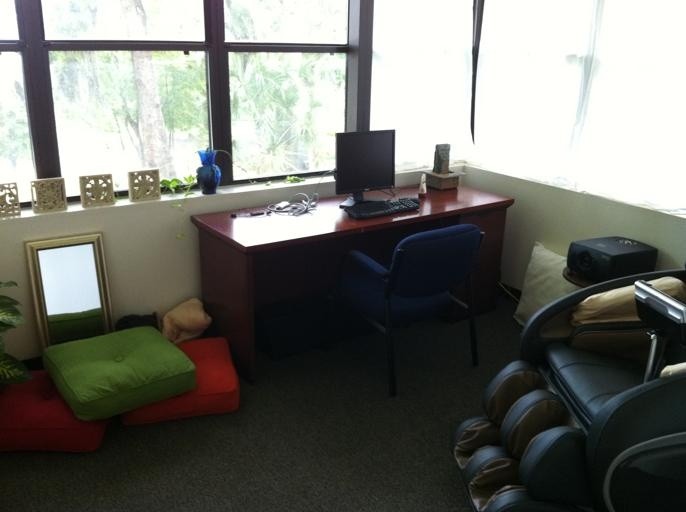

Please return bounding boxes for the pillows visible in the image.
[510,241,584,330]
[1,364,108,455]
[113,336,241,429]
[41,323,200,424]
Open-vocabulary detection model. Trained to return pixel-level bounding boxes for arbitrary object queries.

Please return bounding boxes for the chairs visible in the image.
[449,266,685,508]
[327,222,485,397]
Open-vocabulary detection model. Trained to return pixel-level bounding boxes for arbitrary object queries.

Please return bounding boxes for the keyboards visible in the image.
[345,198,420,220]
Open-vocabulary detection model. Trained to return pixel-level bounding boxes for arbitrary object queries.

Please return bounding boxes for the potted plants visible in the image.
[161,145,233,240]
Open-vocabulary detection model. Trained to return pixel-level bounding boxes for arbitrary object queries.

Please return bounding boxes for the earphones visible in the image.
[311,201,317,207]
[302,199,308,206]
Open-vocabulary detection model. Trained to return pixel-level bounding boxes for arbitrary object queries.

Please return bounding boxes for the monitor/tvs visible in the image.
[336,130,395,207]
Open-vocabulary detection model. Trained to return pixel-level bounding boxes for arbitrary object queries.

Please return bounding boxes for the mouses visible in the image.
[411,198,420,205]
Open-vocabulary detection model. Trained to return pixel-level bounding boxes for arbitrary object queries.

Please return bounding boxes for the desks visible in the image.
[189,180,517,383]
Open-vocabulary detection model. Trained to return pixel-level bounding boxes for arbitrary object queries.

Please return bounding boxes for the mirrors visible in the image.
[21,232,114,354]
